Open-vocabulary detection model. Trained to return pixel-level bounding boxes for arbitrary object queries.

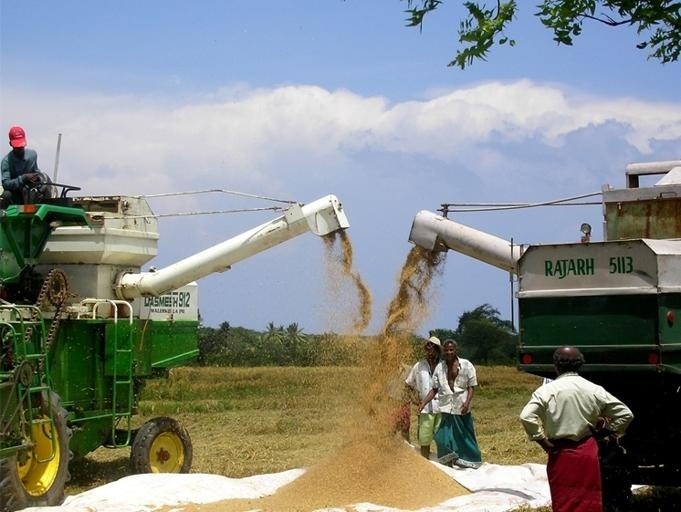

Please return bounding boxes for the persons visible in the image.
[519,346,637,511]
[404,335,443,459]
[396,361,414,444]
[416,338,483,468]
[0,125,53,211]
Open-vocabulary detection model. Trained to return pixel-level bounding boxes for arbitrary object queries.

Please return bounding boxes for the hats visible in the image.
[429,336,441,346]
[8,126,28,149]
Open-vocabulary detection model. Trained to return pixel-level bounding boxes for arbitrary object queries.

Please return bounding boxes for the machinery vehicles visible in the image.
[1,174,353,512]
[403,158,681,511]
